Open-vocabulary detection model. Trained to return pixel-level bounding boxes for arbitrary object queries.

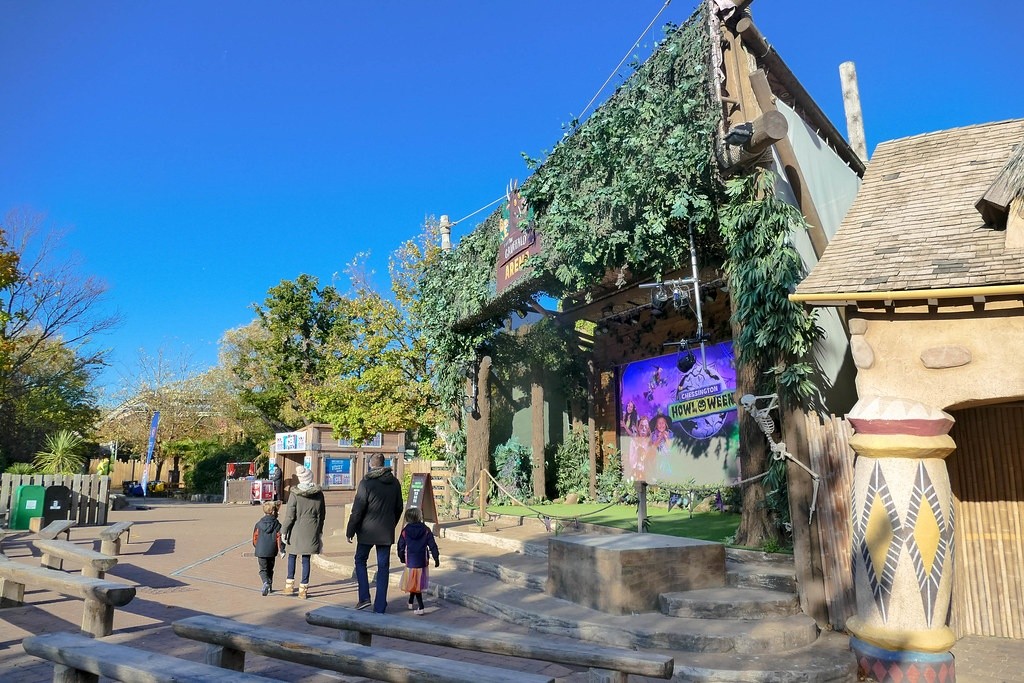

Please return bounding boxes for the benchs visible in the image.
[0,520,676,683]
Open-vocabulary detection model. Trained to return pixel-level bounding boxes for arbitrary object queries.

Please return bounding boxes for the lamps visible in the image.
[678,340,696,373]
[649,283,668,318]
[595,310,639,335]
[699,280,729,303]
[517,304,528,318]
[673,285,692,314]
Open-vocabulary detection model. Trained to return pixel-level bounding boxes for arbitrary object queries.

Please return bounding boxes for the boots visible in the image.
[299,584,308,599]
[284,579,294,594]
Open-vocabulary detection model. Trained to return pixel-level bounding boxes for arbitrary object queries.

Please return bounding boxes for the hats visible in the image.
[296,465,313,485]
[370,453,385,468]
[274,463,278,467]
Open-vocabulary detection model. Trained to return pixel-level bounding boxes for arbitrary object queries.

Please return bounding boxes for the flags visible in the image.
[140,412,159,495]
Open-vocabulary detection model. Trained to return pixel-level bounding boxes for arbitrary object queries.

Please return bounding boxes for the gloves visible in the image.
[433,557,440,567]
[281,533,288,545]
[400,554,406,563]
[279,549,286,559]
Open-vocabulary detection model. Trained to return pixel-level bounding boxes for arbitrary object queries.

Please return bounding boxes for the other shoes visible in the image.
[355,599,371,610]
[373,609,383,615]
[413,608,424,615]
[262,582,272,596]
[406,601,412,610]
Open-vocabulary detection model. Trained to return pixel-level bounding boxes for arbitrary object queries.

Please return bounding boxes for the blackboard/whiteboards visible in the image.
[404,473,440,523]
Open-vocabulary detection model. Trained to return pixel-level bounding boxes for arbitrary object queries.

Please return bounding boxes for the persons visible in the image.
[397,508,440,615]
[280,467,326,601]
[252,502,285,596]
[620,401,673,480]
[345,453,403,613]
[271,464,282,501]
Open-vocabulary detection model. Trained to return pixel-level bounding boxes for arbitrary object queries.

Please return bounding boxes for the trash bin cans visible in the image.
[11,484,71,531]
